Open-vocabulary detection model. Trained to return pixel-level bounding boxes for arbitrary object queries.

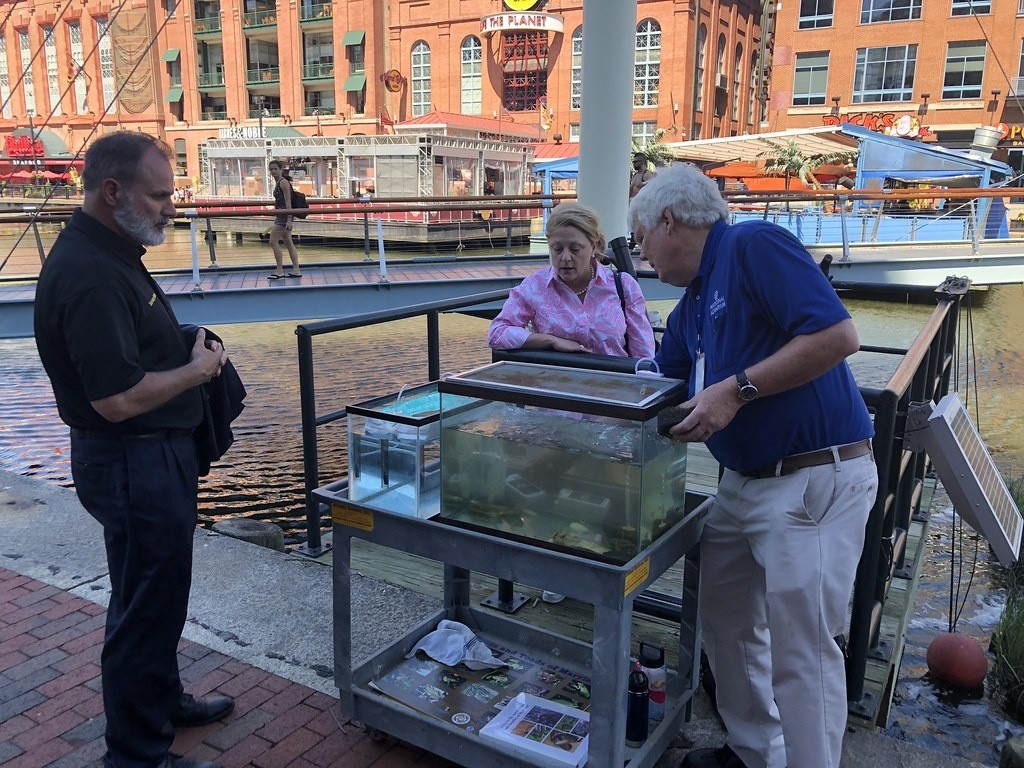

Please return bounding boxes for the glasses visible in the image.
[632,160,643,164]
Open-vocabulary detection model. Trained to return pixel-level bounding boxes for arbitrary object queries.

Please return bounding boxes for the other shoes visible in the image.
[542,590,566,603]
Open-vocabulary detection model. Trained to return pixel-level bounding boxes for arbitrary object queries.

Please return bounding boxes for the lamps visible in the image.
[921,93,930,105]
[281,114,292,123]
[340,113,347,121]
[553,134,562,143]
[991,89,1001,101]
[226,118,238,126]
[832,96,841,106]
[183,119,189,126]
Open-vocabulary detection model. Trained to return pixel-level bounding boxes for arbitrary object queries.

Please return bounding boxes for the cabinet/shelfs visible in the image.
[311,474,712,768]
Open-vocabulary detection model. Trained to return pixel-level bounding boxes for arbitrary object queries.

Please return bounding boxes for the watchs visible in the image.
[736,370,759,402]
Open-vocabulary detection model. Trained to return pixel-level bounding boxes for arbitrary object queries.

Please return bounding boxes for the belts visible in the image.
[741,439,872,479]
[70,427,197,444]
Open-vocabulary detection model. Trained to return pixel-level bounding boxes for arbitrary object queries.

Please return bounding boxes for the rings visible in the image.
[580,345,585,348]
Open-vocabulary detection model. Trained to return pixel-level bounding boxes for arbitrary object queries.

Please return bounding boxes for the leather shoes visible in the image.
[172,693,235,728]
[164,750,224,768]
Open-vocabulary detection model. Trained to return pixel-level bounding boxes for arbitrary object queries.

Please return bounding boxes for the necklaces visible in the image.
[574,265,594,295]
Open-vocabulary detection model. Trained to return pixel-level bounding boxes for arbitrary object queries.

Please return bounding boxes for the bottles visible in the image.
[626,661,648,747]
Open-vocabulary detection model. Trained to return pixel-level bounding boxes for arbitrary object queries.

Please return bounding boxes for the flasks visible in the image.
[633,639,667,719]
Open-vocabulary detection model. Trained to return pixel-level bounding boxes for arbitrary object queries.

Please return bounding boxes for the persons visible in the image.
[262,108,270,116]
[33,133,236,768]
[628,163,877,768]
[488,202,655,360]
[173,184,193,204]
[485,183,495,195]
[267,161,302,279]
[629,152,656,255]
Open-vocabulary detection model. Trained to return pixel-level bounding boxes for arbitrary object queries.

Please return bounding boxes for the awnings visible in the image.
[341,30,365,46]
[161,49,180,62]
[165,89,183,102]
[342,74,366,91]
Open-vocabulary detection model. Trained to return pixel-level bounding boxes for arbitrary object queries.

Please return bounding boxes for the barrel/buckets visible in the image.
[969,126,1004,160]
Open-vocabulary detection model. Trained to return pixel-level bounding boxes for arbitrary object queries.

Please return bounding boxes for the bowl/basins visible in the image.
[549,732,563,740]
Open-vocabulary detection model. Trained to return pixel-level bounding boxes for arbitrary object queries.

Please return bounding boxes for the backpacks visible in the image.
[277,178,309,219]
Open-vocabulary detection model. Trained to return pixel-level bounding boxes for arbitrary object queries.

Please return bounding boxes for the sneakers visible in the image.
[680,742,747,768]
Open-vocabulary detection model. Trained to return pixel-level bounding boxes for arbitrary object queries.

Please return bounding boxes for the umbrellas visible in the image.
[0,169,61,184]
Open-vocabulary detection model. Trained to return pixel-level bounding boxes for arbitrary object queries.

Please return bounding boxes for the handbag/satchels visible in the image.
[613,269,660,356]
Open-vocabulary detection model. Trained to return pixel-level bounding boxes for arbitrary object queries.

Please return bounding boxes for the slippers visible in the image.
[267,274,285,279]
[284,273,302,278]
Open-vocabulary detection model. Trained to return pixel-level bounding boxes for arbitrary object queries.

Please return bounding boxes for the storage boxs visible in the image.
[438,360,690,566]
[344,380,493,520]
[479,692,591,768]
